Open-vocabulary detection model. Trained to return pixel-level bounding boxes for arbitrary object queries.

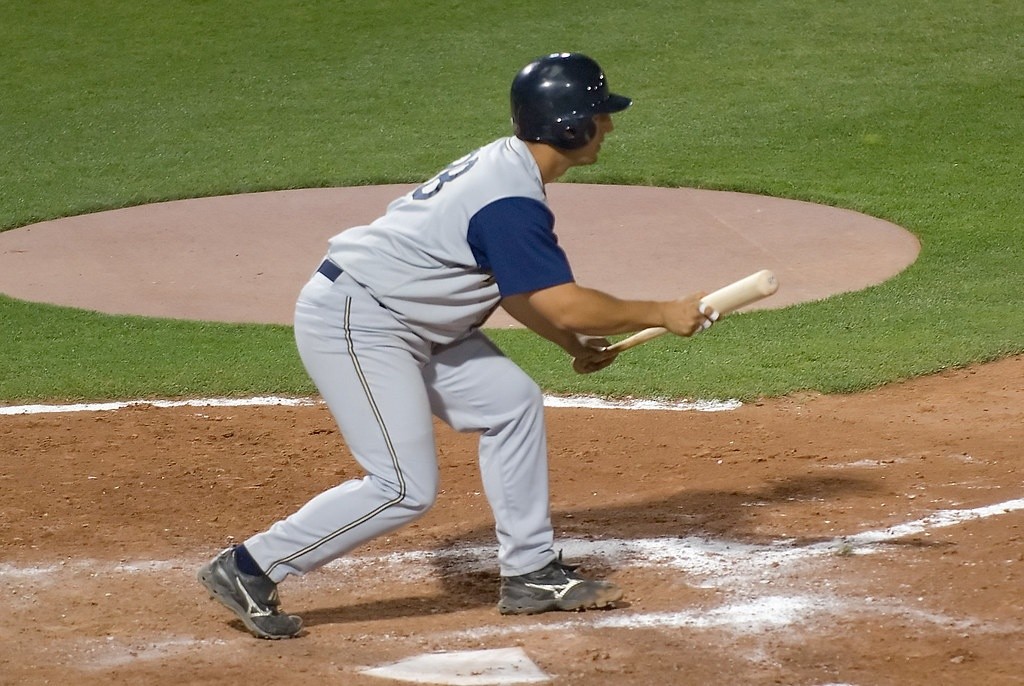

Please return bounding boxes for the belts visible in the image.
[317,259,344,282]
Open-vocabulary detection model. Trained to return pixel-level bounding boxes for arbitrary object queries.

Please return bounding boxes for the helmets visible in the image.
[511,52,632,149]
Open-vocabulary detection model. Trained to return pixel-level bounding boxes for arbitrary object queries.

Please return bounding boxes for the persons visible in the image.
[197,51,722,640]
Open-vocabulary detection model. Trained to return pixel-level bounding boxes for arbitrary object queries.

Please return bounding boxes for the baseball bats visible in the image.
[569,263,781,381]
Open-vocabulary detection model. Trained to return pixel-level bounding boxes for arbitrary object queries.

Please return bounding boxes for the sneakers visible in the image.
[198,546,304,640]
[498,548,623,614]
